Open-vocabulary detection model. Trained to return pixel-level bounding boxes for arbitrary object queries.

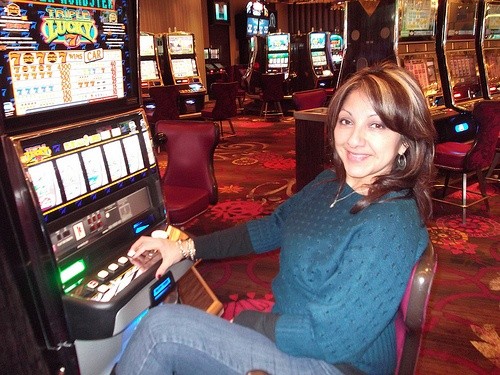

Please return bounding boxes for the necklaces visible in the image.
[330,187,363,208]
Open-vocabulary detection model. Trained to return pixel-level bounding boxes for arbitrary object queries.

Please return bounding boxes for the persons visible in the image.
[114,62,437,375]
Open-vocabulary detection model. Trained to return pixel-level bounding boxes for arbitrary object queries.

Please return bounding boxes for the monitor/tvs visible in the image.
[139,36,155,56]
[141,61,159,80]
[268,34,290,51]
[329,34,344,49]
[267,52,289,67]
[309,33,327,49]
[331,51,344,62]
[168,35,194,54]
[171,58,199,77]
[310,51,328,66]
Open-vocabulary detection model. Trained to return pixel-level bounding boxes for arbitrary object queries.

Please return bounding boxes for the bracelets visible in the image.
[178,238,196,260]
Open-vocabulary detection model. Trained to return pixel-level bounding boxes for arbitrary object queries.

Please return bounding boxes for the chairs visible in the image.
[261,72,285,122]
[292,88,327,111]
[201,81,239,137]
[230,64,247,111]
[245,239,439,375]
[431,100,500,222]
[145,85,179,153]
[151,119,220,225]
